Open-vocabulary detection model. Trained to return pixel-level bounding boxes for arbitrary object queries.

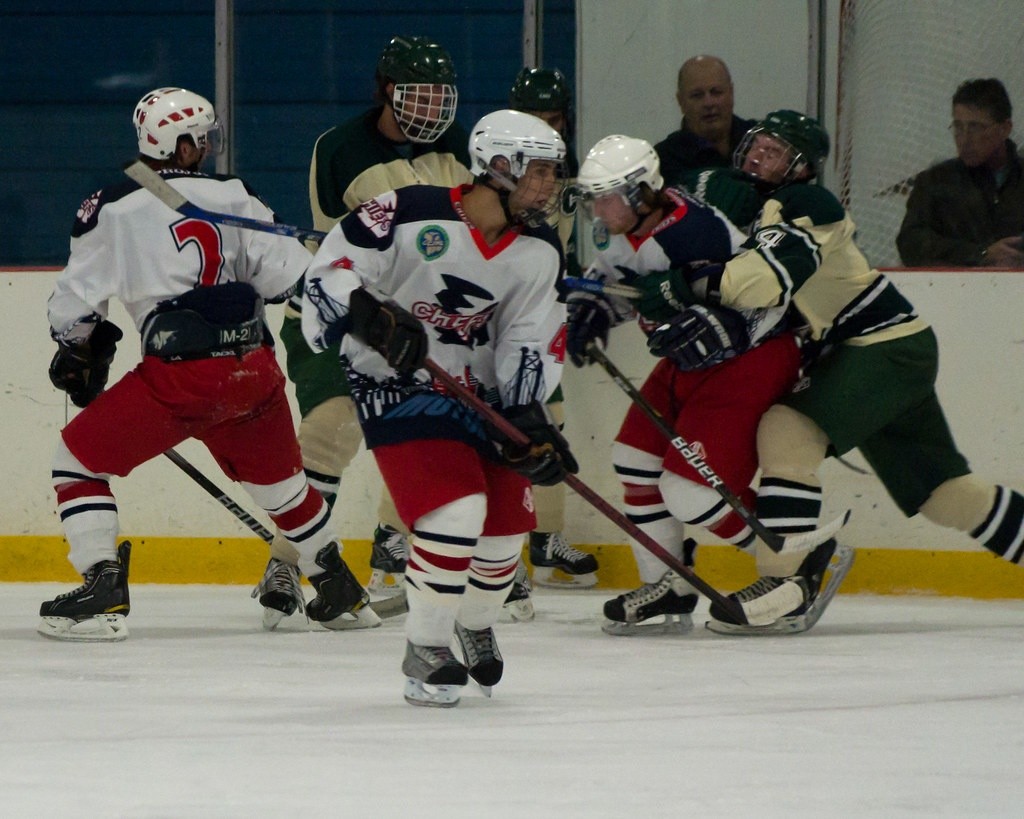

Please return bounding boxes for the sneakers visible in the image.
[365,522,412,593]
[304,540,383,629]
[531,529,600,589]
[499,583,534,623]
[785,537,854,633]
[250,558,306,630]
[454,621,504,698]
[704,575,807,634]
[400,639,468,707]
[603,538,701,636]
[38,540,132,641]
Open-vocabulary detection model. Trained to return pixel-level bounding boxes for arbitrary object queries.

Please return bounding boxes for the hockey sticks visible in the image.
[424,353,805,628]
[587,344,851,558]
[119,153,638,301]
[162,446,410,619]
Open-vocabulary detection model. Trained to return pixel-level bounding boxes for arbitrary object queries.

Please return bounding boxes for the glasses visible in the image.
[947,119,999,136]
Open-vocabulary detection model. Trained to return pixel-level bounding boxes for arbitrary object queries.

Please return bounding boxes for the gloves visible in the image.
[340,288,429,380]
[485,400,578,486]
[48,320,123,408]
[646,299,749,369]
[563,297,616,366]
[624,266,695,321]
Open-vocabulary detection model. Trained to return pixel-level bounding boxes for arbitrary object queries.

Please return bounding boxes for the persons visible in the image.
[32,86,386,643]
[689,109,1023,640]
[649,54,761,193]
[368,60,602,620]
[561,133,857,638]
[296,105,578,710]
[891,77,1023,272]
[249,31,540,630]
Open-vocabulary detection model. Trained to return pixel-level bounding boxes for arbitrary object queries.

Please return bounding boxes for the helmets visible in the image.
[376,33,458,142]
[576,134,664,206]
[468,109,568,232]
[732,108,829,184]
[507,65,572,113]
[133,87,217,161]
